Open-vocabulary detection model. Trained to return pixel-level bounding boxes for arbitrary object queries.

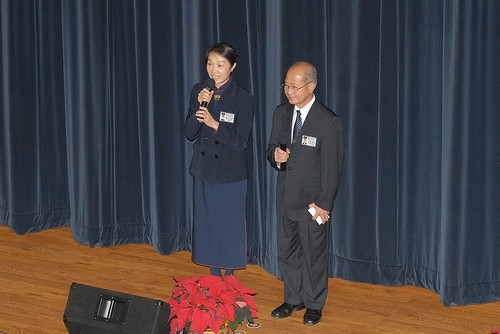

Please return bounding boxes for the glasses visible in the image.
[280,81,315,93]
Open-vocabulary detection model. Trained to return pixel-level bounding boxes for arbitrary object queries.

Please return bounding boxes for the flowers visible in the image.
[166,274,259,334]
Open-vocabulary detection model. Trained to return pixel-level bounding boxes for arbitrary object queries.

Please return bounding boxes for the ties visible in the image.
[292,110,302,143]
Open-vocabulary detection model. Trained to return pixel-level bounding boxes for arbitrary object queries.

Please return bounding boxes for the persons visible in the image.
[182,42,256,276]
[220,113,225,121]
[303,137,308,145]
[264,61,346,327]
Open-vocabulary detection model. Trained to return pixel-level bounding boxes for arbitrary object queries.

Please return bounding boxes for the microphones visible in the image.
[278,131,288,171]
[197,79,215,119]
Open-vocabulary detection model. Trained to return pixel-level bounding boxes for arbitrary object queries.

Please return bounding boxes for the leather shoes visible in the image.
[271,301,306,318]
[303,308,322,325]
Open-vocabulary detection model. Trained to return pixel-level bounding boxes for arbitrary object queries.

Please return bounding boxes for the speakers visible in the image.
[63,282,191,334]
